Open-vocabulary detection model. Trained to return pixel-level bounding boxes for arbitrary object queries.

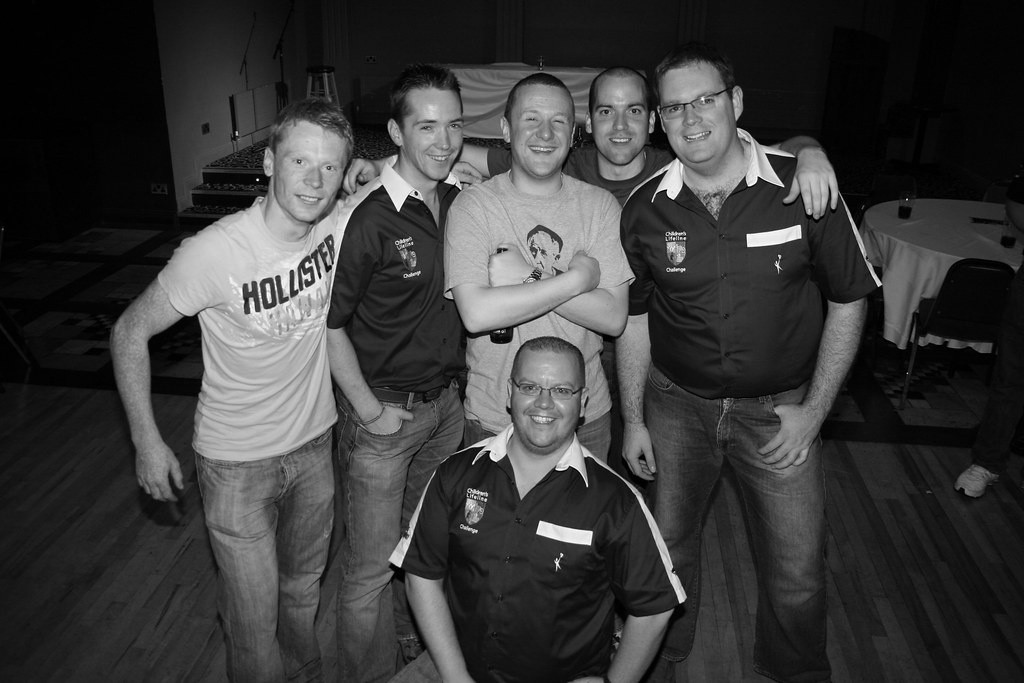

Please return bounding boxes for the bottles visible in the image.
[306,65,341,110]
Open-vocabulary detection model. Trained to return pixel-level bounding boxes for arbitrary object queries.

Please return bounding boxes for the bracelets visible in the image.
[360,402,385,426]
[602,675,611,683]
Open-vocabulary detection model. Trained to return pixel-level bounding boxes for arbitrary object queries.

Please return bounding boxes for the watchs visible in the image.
[523,268,543,285]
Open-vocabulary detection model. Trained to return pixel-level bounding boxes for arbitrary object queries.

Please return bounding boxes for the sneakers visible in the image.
[953,463,1000,499]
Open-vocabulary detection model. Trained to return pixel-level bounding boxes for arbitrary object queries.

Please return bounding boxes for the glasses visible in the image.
[510,377,584,400]
[659,88,733,121]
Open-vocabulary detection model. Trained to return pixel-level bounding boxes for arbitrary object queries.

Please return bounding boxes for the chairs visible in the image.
[898,258,1016,413]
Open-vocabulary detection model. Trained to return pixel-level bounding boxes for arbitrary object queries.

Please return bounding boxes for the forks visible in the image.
[968,216,1009,226]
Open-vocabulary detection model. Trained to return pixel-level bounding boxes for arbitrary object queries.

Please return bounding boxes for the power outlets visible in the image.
[202,123,210,134]
[365,56,376,64]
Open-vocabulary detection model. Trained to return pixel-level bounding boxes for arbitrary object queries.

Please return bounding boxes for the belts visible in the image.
[370,386,445,403]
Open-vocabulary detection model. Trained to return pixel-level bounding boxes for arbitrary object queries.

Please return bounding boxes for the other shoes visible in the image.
[639,654,675,683]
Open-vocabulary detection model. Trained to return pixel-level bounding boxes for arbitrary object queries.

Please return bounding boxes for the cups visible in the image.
[999,222,1017,248]
[898,192,916,220]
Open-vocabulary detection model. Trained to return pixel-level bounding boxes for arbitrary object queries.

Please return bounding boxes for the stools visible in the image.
[305,66,339,110]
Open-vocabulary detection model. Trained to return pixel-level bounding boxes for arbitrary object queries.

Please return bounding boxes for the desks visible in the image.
[859,199,1024,377]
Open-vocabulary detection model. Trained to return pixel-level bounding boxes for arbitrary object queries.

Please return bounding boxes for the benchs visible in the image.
[432,64,647,138]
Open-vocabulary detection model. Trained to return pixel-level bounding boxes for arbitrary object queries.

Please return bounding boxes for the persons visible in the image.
[955,175,1024,499]
[111,43,885,683]
[385,336,690,683]
